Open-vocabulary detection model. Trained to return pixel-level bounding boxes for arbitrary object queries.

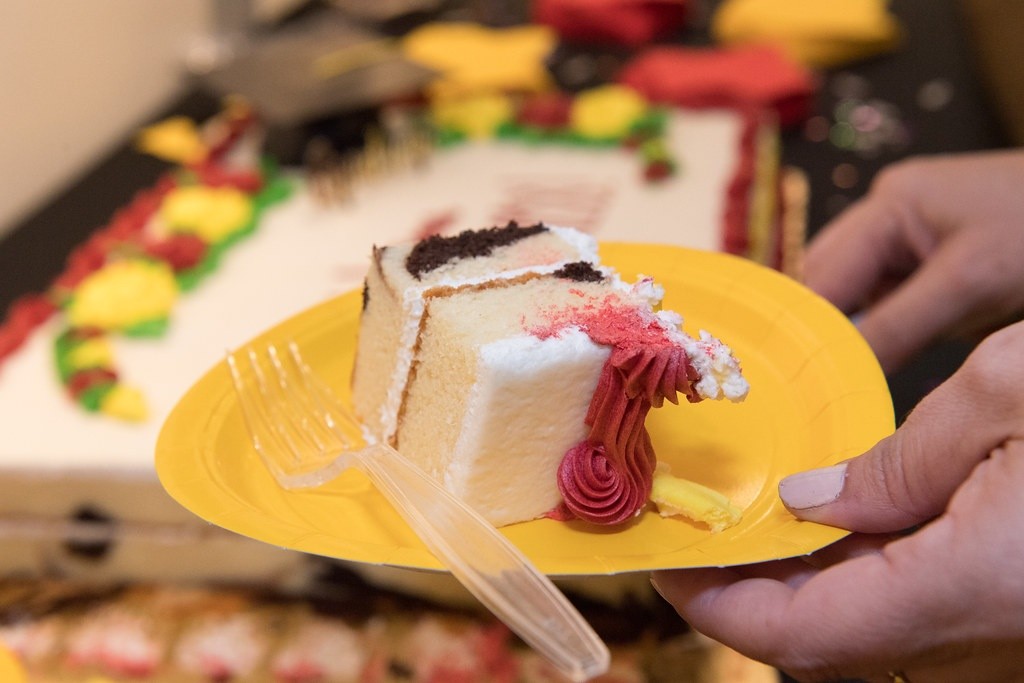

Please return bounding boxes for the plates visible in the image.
[152,241,897,576]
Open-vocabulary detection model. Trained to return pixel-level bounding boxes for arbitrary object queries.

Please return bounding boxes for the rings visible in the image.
[888,671,908,683]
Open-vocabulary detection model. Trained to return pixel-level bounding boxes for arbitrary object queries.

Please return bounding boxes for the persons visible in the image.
[808,0,1024,391]
[649,150,1024,683]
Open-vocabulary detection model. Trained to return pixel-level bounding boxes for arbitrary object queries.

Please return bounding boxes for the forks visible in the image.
[230,342,611,683]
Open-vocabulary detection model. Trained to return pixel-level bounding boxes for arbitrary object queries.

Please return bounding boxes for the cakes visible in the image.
[1,23,785,604]
[348,220,748,529]
